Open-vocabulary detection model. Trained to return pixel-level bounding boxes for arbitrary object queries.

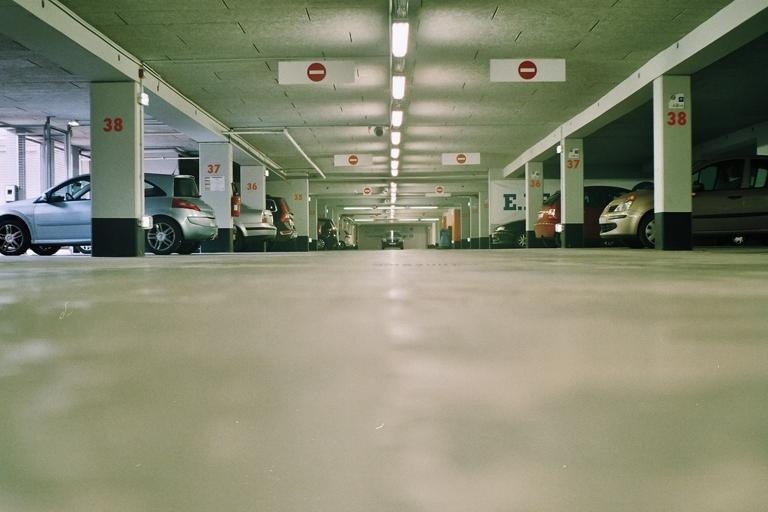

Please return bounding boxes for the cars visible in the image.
[382,230,404,250]
[338,228,357,250]
[598,153,766,250]
[265,194,298,248]
[0,171,220,255]
[232,201,277,250]
[535,184,635,249]
[318,218,339,250]
[490,219,531,246]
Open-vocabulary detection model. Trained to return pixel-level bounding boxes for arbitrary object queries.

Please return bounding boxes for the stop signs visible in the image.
[489,58,565,84]
[277,61,360,85]
[363,187,372,196]
[333,153,371,167]
[441,153,482,167]
[426,185,452,198]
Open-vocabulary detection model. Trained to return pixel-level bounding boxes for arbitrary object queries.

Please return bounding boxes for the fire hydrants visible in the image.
[229,183,241,217]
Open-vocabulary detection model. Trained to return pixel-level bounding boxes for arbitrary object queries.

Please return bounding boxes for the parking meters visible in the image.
[4,185,17,202]
